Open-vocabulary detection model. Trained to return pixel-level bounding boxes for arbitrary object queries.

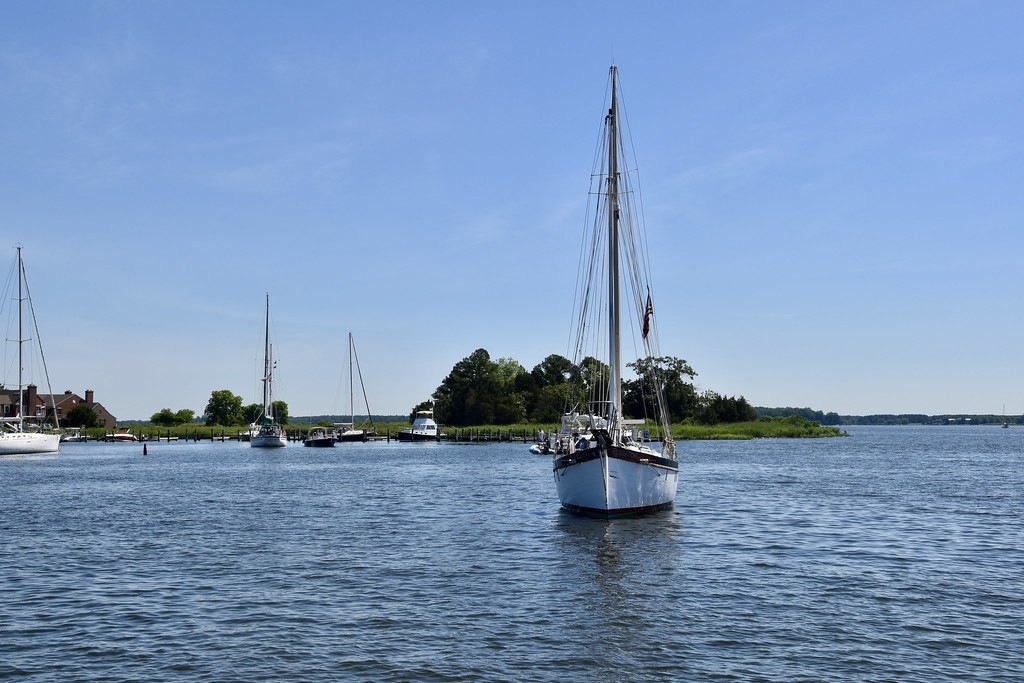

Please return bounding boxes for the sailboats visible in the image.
[551,64,681,517]
[250,295,288,449]
[333,331,379,441]
[0,242,64,455]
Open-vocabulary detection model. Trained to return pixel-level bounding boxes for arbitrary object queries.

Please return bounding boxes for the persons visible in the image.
[643,428,649,438]
[537,427,545,440]
[342,427,345,433]
[554,426,558,434]
[281,426,284,432]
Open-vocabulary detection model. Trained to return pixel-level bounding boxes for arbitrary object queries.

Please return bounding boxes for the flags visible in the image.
[642,296,654,339]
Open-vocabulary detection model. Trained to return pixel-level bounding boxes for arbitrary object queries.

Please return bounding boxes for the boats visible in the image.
[106,427,137,443]
[239,422,259,441]
[529,444,556,455]
[302,426,339,448]
[59,430,91,443]
[397,410,449,441]
